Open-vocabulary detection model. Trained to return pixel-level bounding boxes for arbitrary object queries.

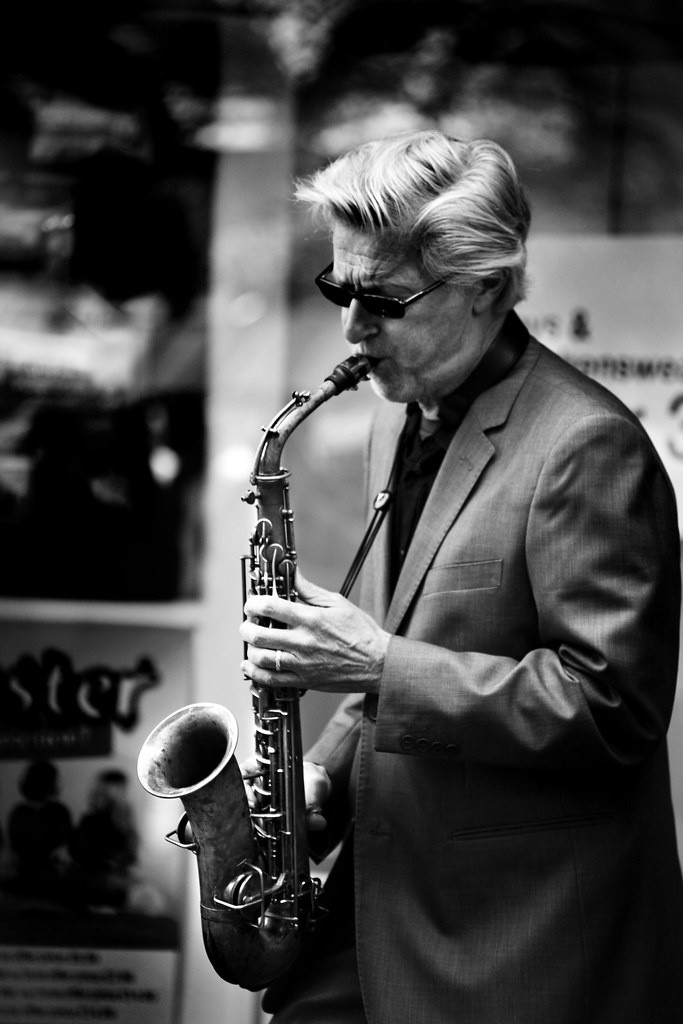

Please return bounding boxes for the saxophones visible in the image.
[142,356,382,993]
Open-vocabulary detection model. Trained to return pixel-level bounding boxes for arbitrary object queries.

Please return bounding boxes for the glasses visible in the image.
[315,261,458,318]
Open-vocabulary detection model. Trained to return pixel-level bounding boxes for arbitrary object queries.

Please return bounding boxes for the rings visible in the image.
[276,649,282,670]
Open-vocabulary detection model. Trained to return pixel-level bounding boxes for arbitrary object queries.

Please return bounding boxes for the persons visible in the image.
[241,127,683,1024]
[9,764,135,911]
